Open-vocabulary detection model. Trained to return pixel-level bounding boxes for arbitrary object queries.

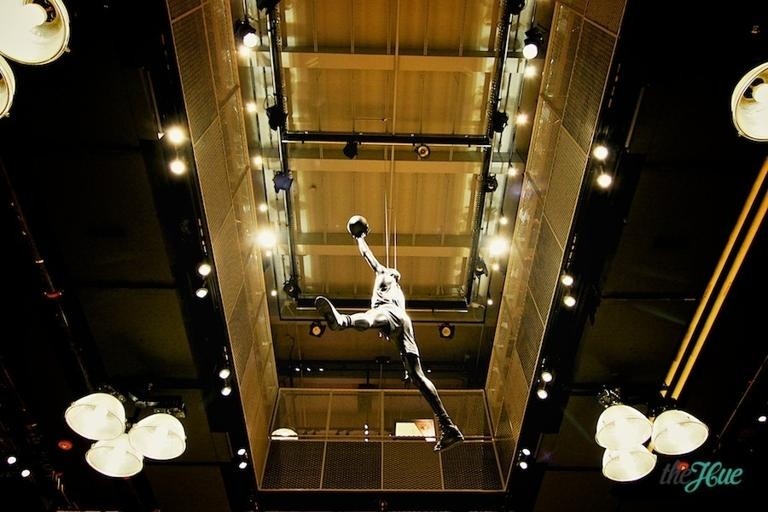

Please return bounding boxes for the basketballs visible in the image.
[347,216,368,236]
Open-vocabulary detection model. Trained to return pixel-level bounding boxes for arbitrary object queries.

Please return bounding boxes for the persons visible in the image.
[314,223,466,454]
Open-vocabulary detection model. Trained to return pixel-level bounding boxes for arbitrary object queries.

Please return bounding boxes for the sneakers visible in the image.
[313,295,346,332]
[430,425,465,455]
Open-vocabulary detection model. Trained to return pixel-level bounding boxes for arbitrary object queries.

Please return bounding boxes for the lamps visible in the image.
[730,60,768,143]
[342,135,431,159]
[0,0,70,121]
[308,321,326,338]
[468,1,529,278]
[594,364,709,482]
[438,323,456,340]
[229,14,300,298]
[64,358,189,478]
